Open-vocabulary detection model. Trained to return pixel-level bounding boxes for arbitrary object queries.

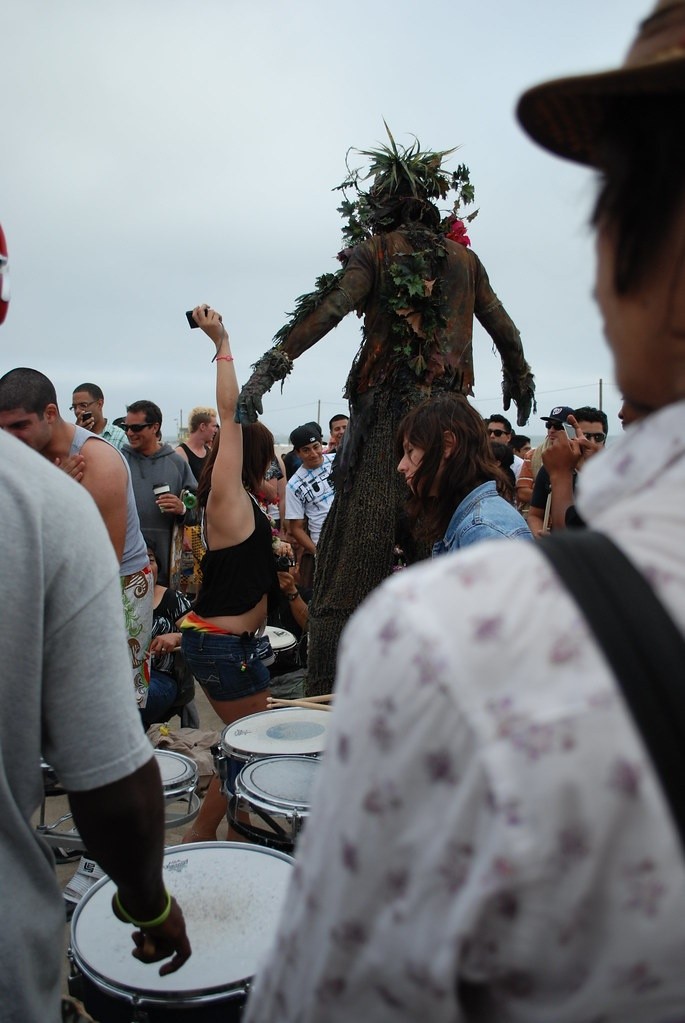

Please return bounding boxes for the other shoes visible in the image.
[51,826,91,864]
[60,855,108,910]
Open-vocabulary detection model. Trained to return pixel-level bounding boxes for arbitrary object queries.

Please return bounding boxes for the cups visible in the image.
[152,481,171,514]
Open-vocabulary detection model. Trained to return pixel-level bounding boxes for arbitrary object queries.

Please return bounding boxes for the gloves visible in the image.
[501,367,535,426]
[232,347,290,427]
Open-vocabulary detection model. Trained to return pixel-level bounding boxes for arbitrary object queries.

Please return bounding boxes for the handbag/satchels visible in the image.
[170,488,208,595]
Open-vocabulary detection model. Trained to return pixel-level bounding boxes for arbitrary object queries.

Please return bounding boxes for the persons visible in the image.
[232,140,542,743]
[397,393,539,564]
[116,400,205,590]
[1,366,653,1023]
[183,305,275,850]
[249,2,685,1023]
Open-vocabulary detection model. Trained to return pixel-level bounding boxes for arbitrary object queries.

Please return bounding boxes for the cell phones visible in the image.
[83,411,91,422]
[562,421,579,443]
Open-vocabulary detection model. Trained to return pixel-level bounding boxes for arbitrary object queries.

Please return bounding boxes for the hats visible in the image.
[356,155,444,228]
[515,0,685,167]
[291,424,321,449]
[540,406,575,422]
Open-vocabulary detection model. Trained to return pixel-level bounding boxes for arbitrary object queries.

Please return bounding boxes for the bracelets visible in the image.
[181,501,186,515]
[215,356,236,361]
[112,885,174,929]
[287,590,299,601]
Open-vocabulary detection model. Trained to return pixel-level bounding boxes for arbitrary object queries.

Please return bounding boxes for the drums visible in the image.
[61,838,297,1022]
[253,625,299,670]
[216,705,333,803]
[228,755,318,853]
[36,748,203,844]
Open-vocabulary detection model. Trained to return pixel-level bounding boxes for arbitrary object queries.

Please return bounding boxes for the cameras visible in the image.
[273,554,296,574]
[186,309,208,329]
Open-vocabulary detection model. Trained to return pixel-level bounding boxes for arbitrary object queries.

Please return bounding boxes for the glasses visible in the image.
[582,432,607,443]
[69,396,101,413]
[485,428,511,437]
[119,423,157,434]
[545,421,566,431]
